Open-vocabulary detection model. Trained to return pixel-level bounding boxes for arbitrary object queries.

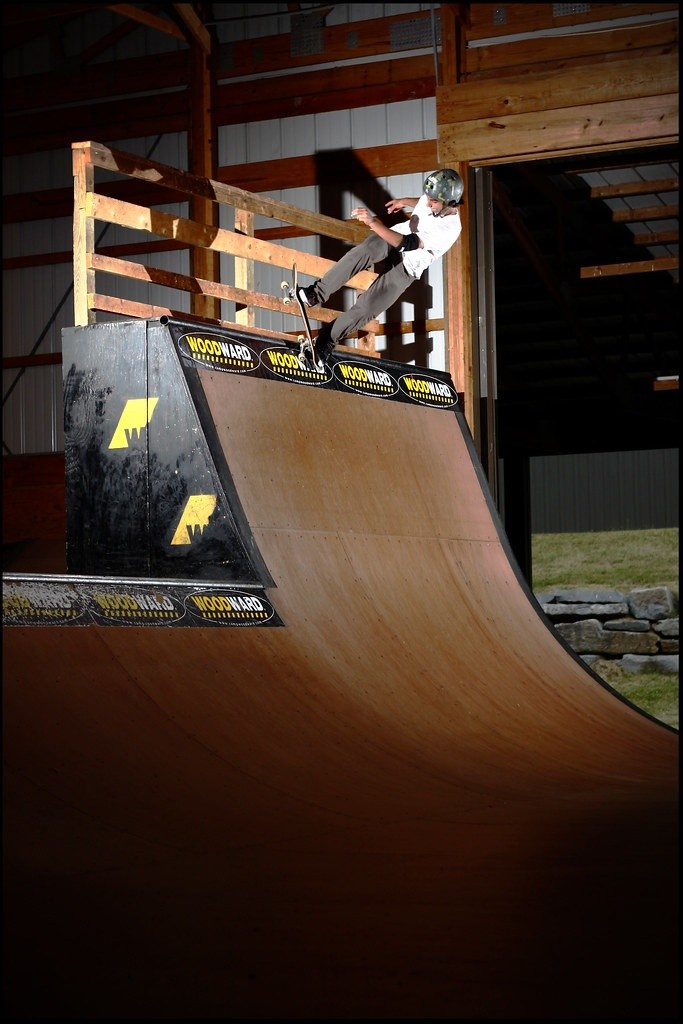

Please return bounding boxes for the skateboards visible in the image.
[281,262,325,374]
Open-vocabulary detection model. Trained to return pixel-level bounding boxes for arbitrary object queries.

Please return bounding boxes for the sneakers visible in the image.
[315,328,333,366]
[299,284,321,308]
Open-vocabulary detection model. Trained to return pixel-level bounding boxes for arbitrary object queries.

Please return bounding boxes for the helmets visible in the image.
[423,168,464,204]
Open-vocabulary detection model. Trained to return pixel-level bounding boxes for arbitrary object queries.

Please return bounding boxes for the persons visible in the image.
[297,168,465,368]
